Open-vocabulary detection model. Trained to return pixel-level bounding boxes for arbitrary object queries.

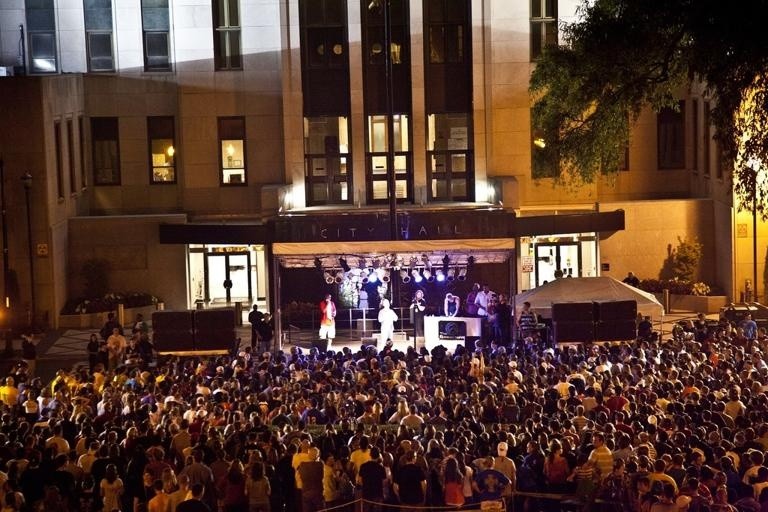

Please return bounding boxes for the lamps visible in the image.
[323,264,468,284]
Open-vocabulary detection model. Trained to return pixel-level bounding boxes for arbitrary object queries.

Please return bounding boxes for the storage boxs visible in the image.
[552,300,637,342]
[152,308,236,351]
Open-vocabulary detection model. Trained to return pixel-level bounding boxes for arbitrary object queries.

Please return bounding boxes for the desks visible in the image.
[424,316,481,358]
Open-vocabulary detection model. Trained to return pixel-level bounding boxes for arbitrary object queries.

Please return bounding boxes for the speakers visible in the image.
[310,340,328,354]
[193,308,236,351]
[552,303,594,343]
[465,336,480,352]
[593,300,637,342]
[152,309,195,352]
[361,337,377,347]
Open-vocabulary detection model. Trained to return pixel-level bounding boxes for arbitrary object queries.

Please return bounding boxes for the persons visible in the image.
[410,289,426,338]
[444,284,513,347]
[623,272,639,288]
[319,294,336,350]
[378,299,398,350]
[0,312,768,512]
[515,302,538,339]
[249,304,264,349]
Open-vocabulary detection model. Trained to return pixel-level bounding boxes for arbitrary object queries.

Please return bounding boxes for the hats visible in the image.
[0,327,768,458]
